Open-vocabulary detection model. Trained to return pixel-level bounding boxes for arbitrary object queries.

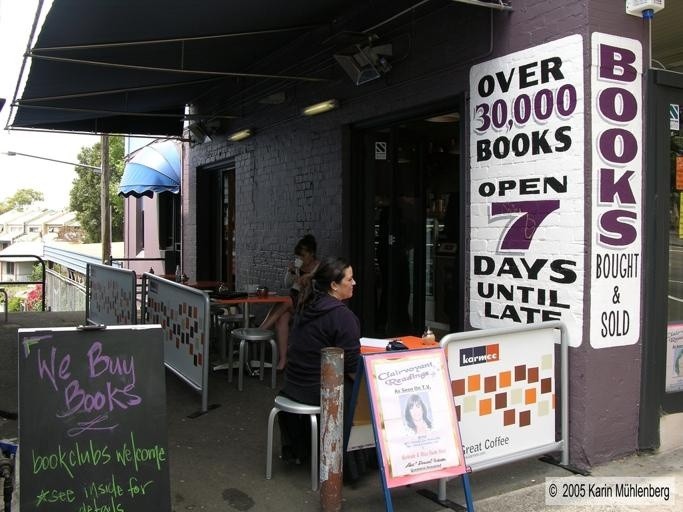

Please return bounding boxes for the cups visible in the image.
[294,259,303,268]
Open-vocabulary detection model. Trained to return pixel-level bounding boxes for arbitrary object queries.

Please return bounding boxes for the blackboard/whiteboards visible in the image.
[19,328,172,512]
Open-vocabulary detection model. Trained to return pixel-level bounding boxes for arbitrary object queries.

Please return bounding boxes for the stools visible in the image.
[265,394,319,494]
[211,309,278,391]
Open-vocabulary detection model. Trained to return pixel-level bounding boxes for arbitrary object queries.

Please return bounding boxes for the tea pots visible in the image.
[219,284,229,295]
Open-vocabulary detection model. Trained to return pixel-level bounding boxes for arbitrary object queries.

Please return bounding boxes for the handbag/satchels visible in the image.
[208,291,248,300]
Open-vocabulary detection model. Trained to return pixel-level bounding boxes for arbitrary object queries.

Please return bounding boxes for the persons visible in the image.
[256,234,325,372]
[275,255,362,486]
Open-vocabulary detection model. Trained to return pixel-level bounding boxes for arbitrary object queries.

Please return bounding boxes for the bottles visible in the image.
[174,265,181,283]
[422,326,435,346]
[256,286,267,297]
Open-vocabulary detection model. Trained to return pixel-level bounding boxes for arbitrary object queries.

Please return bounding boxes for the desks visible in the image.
[135,273,288,373]
[357,334,442,355]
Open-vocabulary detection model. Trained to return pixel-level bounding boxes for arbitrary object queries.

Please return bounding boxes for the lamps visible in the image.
[333,31,394,87]
